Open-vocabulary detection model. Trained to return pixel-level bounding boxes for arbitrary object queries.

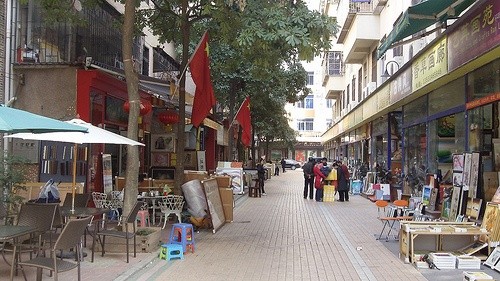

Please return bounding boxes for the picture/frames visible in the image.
[150,133,176,153]
[437,102,495,218]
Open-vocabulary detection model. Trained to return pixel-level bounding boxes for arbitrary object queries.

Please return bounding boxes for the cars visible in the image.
[279,160,301,170]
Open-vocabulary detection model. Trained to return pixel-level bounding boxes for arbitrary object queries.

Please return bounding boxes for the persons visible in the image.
[257,158,267,196]
[302,157,350,202]
[281,159,286,172]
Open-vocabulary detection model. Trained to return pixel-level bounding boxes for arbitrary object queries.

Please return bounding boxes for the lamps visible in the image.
[379,60,399,77]
[435,16,460,29]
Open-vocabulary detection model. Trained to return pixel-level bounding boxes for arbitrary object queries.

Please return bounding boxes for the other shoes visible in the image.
[262,193,267,196]
[337,199,343,201]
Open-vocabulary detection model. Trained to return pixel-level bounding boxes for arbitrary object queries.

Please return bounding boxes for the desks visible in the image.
[408,225,491,263]
[55,205,107,259]
[0,226,40,281]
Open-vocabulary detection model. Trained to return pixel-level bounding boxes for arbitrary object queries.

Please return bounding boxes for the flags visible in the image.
[189,34,216,128]
[235,97,251,147]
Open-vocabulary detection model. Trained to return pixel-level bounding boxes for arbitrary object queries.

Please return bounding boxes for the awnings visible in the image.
[378,0,476,58]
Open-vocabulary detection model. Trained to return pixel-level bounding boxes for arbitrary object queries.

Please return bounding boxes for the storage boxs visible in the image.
[184,169,234,221]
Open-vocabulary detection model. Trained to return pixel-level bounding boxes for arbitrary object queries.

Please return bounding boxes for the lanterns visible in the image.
[123,98,152,124]
[158,110,177,130]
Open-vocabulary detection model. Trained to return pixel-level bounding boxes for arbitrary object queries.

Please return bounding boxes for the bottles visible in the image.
[419,203,423,210]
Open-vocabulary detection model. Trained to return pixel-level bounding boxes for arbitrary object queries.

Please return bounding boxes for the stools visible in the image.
[159,243,183,261]
[167,222,195,254]
[137,209,152,227]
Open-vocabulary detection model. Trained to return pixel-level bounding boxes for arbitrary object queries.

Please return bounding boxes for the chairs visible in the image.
[374,199,415,242]
[248,178,261,198]
[6,188,186,281]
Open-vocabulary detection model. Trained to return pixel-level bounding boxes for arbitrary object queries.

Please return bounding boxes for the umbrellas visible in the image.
[3,118,145,214]
[0,104,88,229]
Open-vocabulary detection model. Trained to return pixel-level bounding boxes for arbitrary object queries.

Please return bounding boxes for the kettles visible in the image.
[155,137,165,149]
[149,192,155,197]
